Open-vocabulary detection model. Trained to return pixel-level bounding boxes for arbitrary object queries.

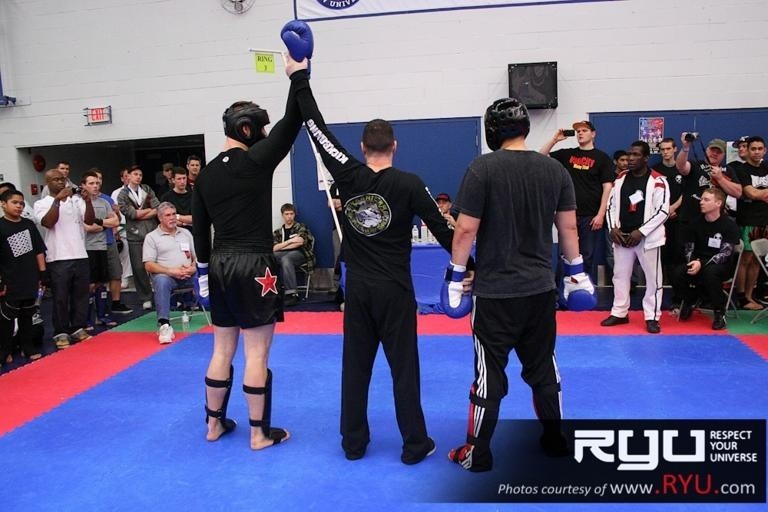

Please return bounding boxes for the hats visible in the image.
[163,163,173,170]
[732,136,750,148]
[572,121,595,131]
[437,194,449,201]
[709,139,726,153]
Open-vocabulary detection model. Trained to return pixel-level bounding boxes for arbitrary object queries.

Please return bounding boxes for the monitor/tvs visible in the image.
[508,62,559,111]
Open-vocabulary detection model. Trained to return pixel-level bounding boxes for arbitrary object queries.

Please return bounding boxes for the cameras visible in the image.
[685,132,699,142]
[563,129,575,137]
[72,187,82,193]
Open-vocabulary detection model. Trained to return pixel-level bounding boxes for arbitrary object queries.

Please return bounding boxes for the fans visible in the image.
[219,0,258,15]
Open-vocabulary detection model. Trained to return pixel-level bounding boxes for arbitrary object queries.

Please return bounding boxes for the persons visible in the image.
[0,154,203,365]
[325,180,348,306]
[272,203,317,307]
[190,20,314,452]
[284,51,477,465]
[434,192,459,228]
[538,120,768,336]
[440,97,598,475]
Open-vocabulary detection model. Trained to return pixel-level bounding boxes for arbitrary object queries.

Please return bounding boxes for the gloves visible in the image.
[192,258,211,310]
[558,254,598,310]
[280,20,313,79]
[440,260,472,317]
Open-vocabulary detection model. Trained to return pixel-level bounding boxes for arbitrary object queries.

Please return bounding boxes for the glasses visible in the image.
[165,168,172,172]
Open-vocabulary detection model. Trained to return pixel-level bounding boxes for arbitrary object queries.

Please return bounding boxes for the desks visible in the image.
[408,240,475,303]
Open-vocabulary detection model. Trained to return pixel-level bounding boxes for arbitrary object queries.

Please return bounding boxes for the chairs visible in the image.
[750,238,768,326]
[276,234,315,300]
[678,235,743,328]
[145,263,212,328]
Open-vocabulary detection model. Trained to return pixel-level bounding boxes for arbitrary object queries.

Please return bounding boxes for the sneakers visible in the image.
[111,304,134,314]
[424,438,436,458]
[752,295,768,305]
[284,293,299,306]
[52,333,70,349]
[449,448,485,471]
[104,306,111,316]
[712,308,727,330]
[70,328,92,342]
[680,301,692,320]
[183,302,200,310]
[158,324,175,343]
[601,316,628,326]
[143,301,152,310]
[646,320,660,333]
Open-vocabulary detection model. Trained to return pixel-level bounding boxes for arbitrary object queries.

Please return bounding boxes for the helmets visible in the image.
[223,101,270,149]
[483,98,530,151]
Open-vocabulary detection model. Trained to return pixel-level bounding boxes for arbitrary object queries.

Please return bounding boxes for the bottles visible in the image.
[411,225,419,244]
